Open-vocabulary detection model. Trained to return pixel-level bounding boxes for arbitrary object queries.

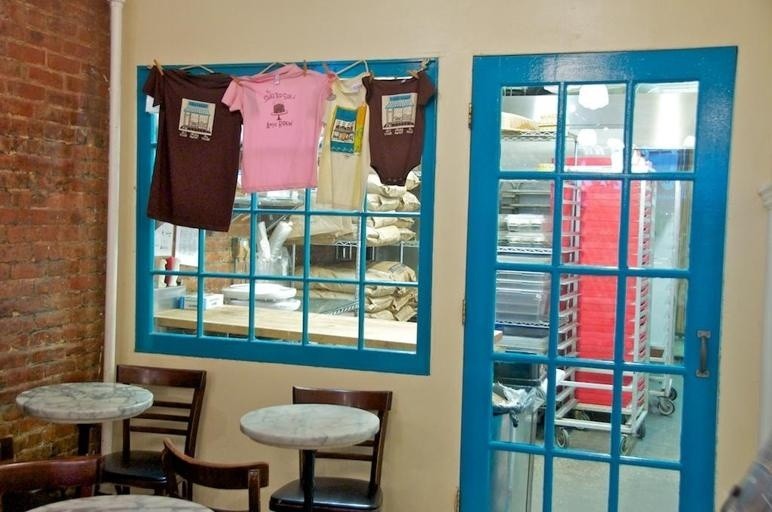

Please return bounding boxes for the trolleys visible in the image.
[649,178,681,417]
[554,166,658,457]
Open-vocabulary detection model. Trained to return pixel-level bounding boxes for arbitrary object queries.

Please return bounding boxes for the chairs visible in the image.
[269,385,394,512]
[0,454,103,512]
[97,363,207,495]
[161,436,270,511]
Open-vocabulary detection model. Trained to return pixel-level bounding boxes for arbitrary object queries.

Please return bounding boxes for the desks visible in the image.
[155,303,505,355]
[23,494,212,512]
[16,381,154,496]
[240,403,380,511]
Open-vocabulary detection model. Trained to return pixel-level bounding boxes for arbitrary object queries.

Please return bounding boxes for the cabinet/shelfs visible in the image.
[272,225,419,289]
